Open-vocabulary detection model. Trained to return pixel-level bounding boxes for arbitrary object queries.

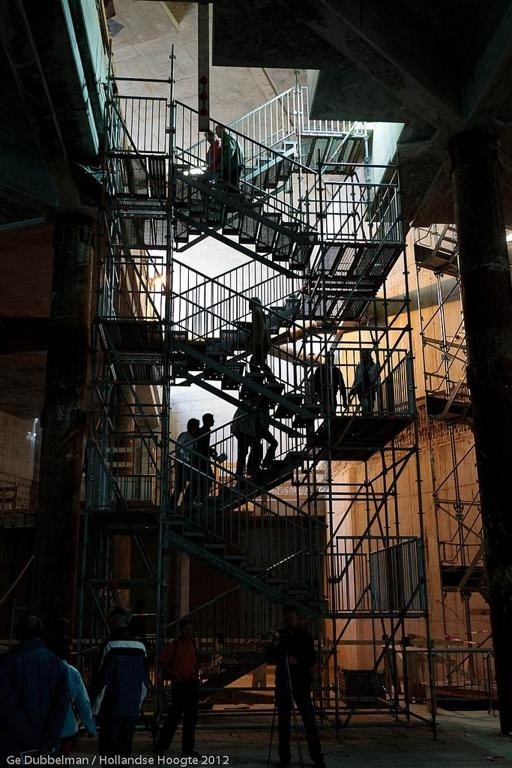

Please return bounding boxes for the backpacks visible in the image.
[161,640,177,680]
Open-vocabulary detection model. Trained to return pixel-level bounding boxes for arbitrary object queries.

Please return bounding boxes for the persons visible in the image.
[312,351,347,420]
[0,615,202,768]
[254,605,327,768]
[215,125,244,194]
[349,349,382,417]
[197,128,221,202]
[256,394,279,469]
[248,296,280,383]
[170,417,201,511]
[196,412,218,498]
[229,394,263,481]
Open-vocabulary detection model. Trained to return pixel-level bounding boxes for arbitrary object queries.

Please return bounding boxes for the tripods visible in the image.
[266,647,305,767]
